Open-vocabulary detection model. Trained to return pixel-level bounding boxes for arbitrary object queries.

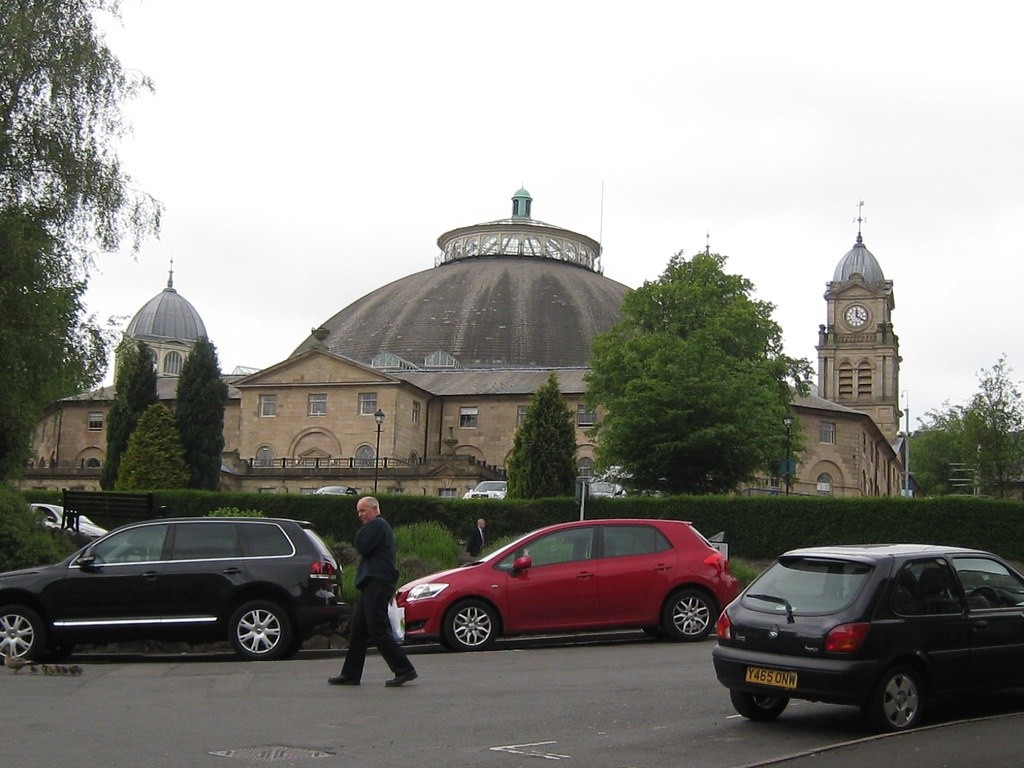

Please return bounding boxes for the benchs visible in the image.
[60,488,168,533]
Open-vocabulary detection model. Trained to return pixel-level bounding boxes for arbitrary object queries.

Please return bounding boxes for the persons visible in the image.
[466,518,485,557]
[328,497,418,688]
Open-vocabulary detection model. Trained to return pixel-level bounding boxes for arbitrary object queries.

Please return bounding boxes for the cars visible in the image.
[461,479,507,501]
[30,503,111,539]
[311,486,358,496]
[590,482,627,500]
[392,517,741,653]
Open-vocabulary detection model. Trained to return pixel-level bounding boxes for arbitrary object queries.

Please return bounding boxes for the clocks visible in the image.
[845,306,869,327]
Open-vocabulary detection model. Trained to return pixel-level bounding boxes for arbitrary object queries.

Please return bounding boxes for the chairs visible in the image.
[849,568,867,594]
[920,568,950,598]
[604,537,616,558]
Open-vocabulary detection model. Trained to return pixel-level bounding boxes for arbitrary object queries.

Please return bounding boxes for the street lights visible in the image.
[374,409,386,495]
[784,410,794,496]
[901,389,909,498]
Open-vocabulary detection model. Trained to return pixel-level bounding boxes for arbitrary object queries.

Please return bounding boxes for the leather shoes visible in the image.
[328,675,360,685]
[386,669,417,687]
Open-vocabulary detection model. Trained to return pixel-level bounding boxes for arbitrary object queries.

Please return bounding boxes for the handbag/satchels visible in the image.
[388,598,405,641]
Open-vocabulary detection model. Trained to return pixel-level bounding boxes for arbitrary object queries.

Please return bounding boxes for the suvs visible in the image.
[711,542,1024,734]
[0,516,350,668]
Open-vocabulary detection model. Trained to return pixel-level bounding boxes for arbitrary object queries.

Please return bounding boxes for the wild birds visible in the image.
[3,645,32,675]
[29,664,81,678]
